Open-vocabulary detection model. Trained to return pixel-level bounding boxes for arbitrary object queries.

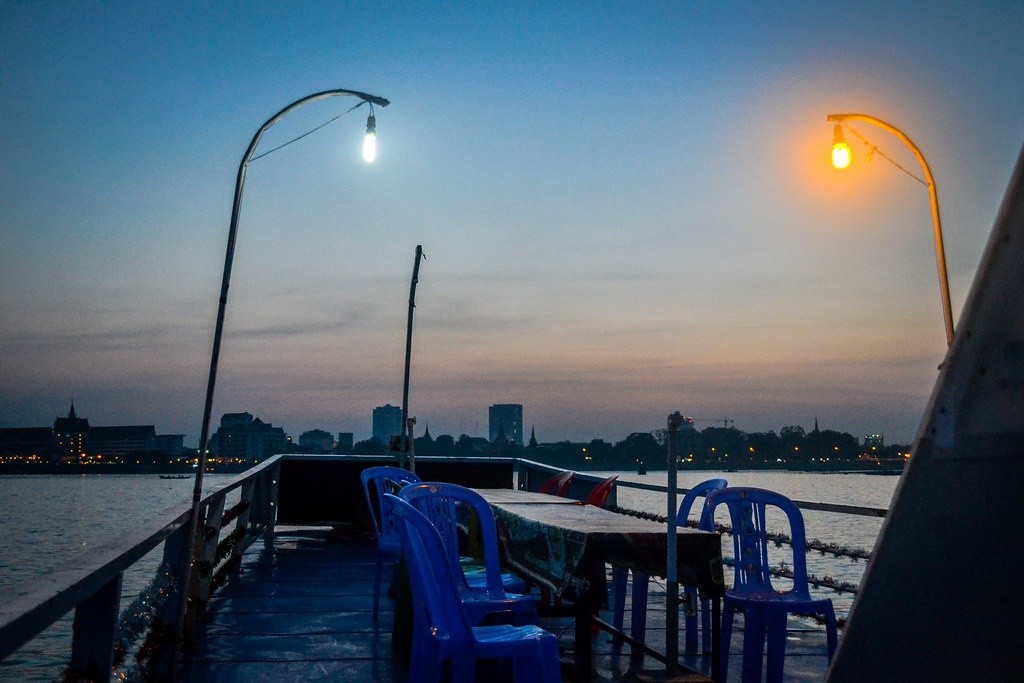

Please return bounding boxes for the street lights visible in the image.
[170,88,391,683]
[824,112,956,351]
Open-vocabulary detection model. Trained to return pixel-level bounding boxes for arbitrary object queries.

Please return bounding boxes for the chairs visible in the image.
[415,496,527,625]
[360,466,421,623]
[539,470,575,497]
[385,477,475,566]
[613,478,727,657]
[705,487,838,683]
[384,492,559,682]
[541,473,619,632]
[398,482,537,627]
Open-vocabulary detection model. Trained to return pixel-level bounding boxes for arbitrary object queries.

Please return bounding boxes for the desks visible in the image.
[466,488,580,556]
[489,502,724,679]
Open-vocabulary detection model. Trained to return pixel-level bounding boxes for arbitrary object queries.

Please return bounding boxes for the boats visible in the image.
[637,466,647,475]
[159,474,191,479]
